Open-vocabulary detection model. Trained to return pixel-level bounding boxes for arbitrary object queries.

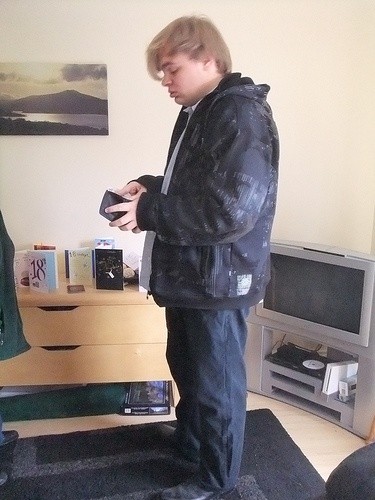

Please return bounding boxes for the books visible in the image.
[12,237,129,292]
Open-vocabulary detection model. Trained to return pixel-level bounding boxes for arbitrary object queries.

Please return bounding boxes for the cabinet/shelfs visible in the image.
[239,298,374,439]
[1,274,182,406]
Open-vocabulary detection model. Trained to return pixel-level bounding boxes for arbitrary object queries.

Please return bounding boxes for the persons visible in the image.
[0,210,33,482]
[105,15,280,500]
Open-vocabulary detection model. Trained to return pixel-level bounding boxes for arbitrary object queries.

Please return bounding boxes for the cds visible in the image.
[302,360,324,370]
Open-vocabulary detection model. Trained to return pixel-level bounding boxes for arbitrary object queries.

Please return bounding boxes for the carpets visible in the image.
[1,408,329,500]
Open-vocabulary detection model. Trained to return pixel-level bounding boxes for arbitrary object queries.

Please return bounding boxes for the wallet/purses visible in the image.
[99,190,137,230]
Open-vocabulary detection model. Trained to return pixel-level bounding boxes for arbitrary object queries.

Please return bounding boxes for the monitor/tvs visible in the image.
[256,240,375,348]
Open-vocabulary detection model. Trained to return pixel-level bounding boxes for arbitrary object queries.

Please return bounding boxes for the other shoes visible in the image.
[151,449,201,474]
[0,471,8,487]
[160,474,237,500]
[0,431,19,447]
